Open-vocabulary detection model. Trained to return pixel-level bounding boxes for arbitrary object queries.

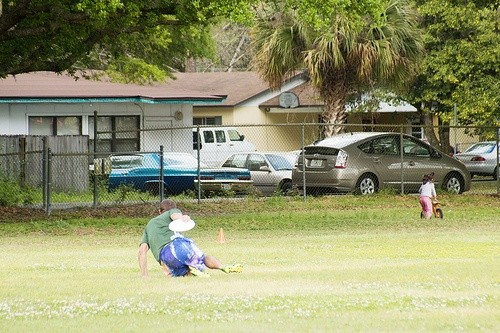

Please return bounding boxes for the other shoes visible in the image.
[190,268,210,279]
[222,264,243,273]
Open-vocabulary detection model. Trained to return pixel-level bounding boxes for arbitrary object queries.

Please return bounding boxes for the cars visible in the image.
[293,131,472,198]
[103,151,255,199]
[378,138,462,156]
[452,141,500,182]
[213,151,295,197]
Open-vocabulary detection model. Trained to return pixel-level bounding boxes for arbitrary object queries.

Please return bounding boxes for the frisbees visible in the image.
[169,219,195,232]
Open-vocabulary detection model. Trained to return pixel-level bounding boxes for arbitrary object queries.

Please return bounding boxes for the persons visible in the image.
[138,199,246,281]
[418,174,438,220]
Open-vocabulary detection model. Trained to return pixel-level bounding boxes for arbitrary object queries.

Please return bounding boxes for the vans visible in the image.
[192,125,260,168]
